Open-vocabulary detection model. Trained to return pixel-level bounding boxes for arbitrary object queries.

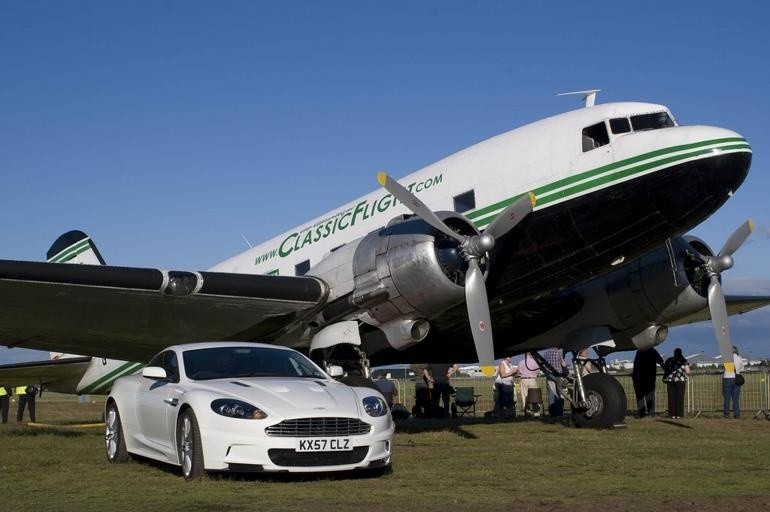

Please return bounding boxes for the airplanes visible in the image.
[1,89,770,427]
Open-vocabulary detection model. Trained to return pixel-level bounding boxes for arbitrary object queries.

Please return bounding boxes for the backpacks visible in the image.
[734,373,745,386]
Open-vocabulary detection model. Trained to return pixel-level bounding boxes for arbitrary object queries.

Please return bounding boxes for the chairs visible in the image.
[523,388,545,417]
[452,387,482,420]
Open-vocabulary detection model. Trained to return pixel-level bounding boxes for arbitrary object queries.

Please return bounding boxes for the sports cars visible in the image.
[105,341,397,480]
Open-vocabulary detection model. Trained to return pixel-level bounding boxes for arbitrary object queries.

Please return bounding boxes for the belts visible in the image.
[520,376,537,380]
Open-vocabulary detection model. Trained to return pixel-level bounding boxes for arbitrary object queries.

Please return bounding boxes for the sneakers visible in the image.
[636,413,741,420]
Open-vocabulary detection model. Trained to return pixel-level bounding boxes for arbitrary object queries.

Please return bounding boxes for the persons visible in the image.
[515,352,546,412]
[630,345,665,419]
[540,345,568,417]
[576,348,592,377]
[422,363,454,418]
[0,386,11,423]
[721,345,745,418]
[410,364,431,418]
[663,347,691,419]
[14,386,38,423]
[490,357,518,419]
[373,369,398,396]
